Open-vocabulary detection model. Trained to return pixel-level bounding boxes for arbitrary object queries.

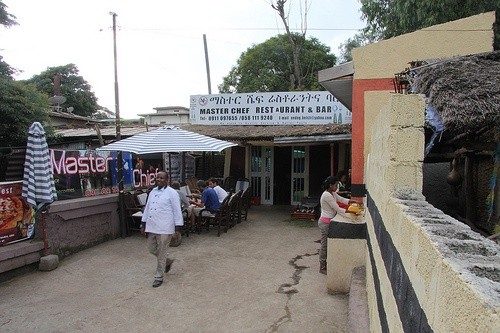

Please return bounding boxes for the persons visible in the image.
[140,171,184,287]
[189,180,220,232]
[172,182,191,220]
[335,171,348,193]
[208,178,232,208]
[318,176,364,273]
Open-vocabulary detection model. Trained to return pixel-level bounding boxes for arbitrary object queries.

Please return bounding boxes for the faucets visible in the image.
[357,201,363,206]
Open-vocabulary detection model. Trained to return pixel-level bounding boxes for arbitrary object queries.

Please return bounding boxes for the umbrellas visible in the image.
[20,122,58,256]
[95,124,239,187]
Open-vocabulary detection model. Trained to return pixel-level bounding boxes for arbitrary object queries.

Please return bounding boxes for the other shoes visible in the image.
[152,280,162,287]
[165,258,174,272]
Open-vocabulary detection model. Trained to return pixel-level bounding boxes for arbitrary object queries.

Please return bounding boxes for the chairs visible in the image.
[175,209,189,238]
[228,189,244,229]
[224,176,237,192]
[123,192,148,239]
[185,179,201,194]
[197,192,233,236]
[236,186,252,224]
[134,189,148,208]
[235,178,250,208]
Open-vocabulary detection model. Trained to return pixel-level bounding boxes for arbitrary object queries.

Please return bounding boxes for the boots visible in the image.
[320,260,327,275]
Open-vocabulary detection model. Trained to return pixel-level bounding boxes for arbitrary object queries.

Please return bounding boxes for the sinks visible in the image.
[329,210,366,238]
